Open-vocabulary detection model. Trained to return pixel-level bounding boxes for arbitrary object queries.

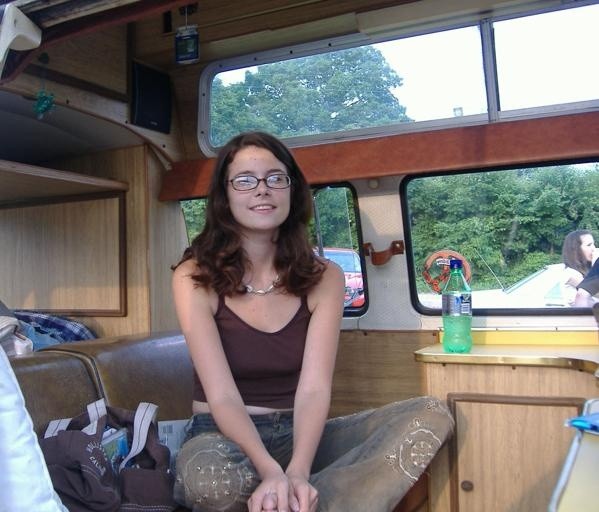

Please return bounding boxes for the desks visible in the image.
[548,395,597,512]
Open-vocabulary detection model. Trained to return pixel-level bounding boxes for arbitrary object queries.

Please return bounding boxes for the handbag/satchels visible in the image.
[39,395,175,512]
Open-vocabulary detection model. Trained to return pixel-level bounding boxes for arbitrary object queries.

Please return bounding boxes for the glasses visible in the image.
[226,171,292,191]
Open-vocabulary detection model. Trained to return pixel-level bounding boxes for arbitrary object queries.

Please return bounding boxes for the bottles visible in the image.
[439,259,475,355]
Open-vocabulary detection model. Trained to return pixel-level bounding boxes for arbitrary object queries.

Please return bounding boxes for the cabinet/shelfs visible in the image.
[411,337,598,512]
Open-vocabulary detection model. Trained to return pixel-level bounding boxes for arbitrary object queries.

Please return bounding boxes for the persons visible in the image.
[169,131,458,512]
[548,228,599,308]
[573,257,599,308]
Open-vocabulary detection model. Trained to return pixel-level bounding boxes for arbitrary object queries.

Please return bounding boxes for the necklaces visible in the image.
[239,270,281,297]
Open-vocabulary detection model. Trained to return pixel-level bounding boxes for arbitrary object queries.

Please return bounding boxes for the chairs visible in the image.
[7,349,98,436]
[36,330,196,421]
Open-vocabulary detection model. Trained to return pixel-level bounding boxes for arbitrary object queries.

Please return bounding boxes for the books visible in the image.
[103,425,135,472]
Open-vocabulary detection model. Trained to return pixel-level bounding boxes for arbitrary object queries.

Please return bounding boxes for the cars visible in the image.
[312,248,367,310]
[421,263,585,310]
